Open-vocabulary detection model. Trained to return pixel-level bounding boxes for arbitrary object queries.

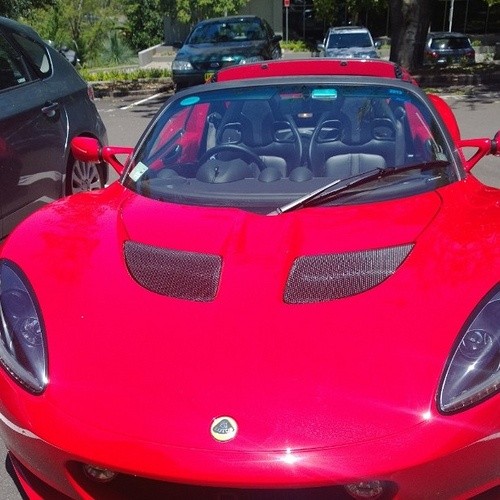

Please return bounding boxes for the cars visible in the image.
[318,26,383,60]
[422,32,477,65]
[168,16,283,95]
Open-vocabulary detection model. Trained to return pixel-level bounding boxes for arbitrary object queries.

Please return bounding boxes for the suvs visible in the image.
[0,18,109,244]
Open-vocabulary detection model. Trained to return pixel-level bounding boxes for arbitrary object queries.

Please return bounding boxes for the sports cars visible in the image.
[0,58,500,498]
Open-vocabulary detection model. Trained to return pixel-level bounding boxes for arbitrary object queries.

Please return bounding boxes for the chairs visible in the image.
[215,99,303,169]
[308,95,406,179]
